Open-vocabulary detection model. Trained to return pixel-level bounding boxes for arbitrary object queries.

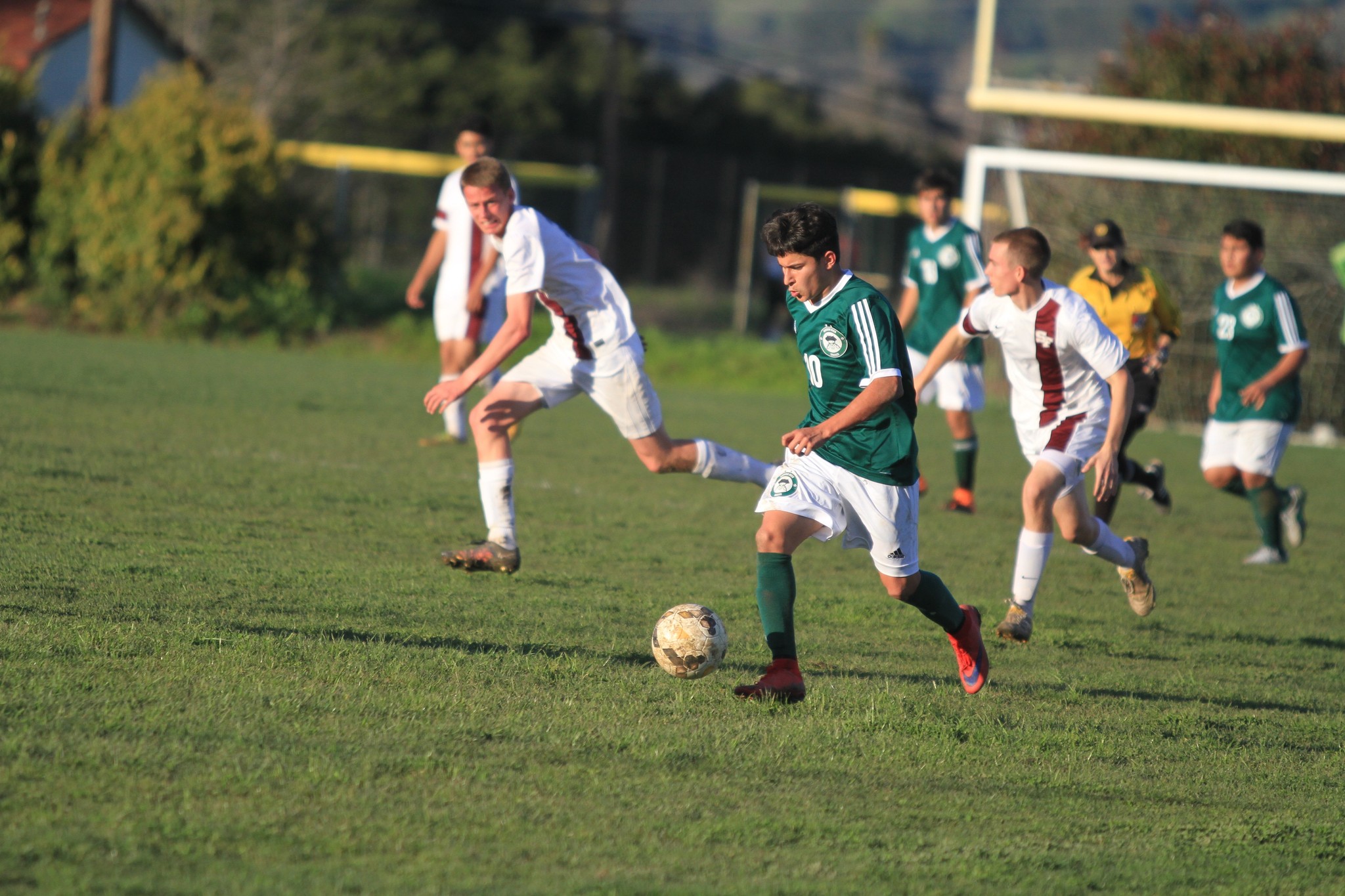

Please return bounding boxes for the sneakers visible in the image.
[734,659,806,704]
[441,540,521,575]
[946,604,989,695]
[1116,537,1157,617]
[995,599,1033,643]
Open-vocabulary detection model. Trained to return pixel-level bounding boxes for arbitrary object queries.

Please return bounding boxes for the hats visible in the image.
[1087,219,1124,249]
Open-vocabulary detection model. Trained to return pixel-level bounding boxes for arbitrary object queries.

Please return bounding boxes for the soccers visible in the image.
[651,602,730,679]
[1311,421,1337,447]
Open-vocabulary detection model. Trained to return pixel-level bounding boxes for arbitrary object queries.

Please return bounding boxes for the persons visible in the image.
[733,205,988,704]
[406,121,522,446]
[891,165,985,513]
[912,228,1157,643]
[1066,220,1183,526]
[1197,217,1308,566]
[423,156,784,577]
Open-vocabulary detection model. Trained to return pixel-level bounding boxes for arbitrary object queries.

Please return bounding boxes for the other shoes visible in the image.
[919,476,928,496]
[1143,459,1172,516]
[420,433,464,446]
[1280,484,1306,548]
[508,418,526,443]
[945,489,975,514]
[1244,547,1288,565]
